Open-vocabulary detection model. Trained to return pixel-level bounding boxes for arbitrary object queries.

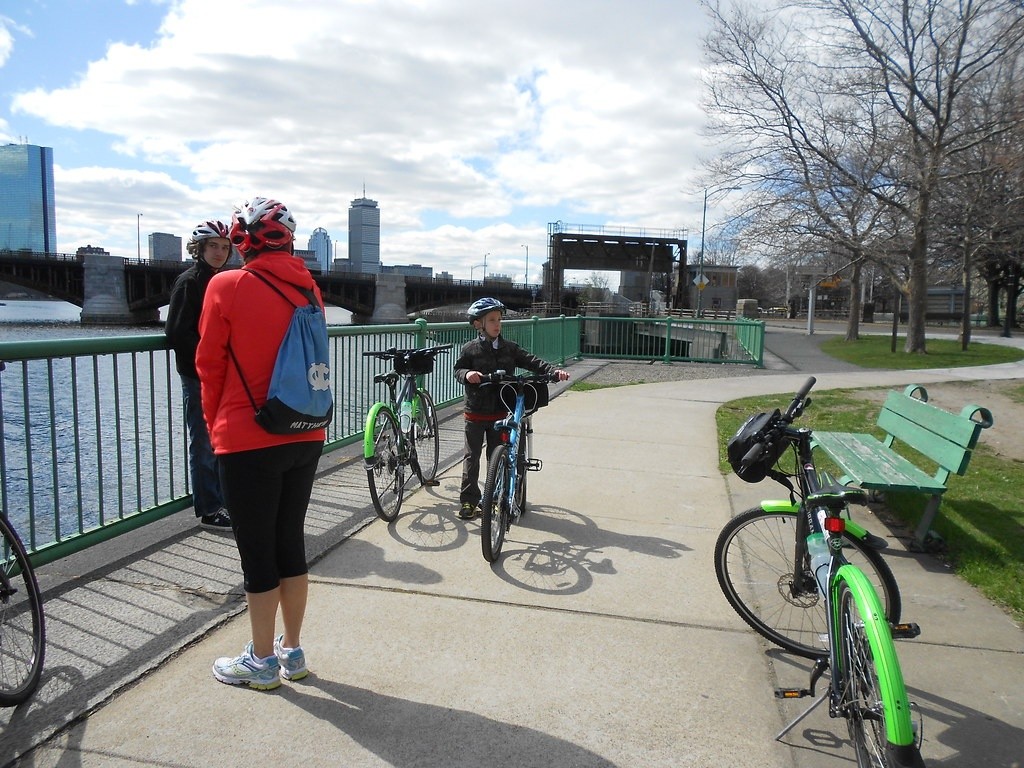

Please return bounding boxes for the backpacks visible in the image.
[230,269,335,433]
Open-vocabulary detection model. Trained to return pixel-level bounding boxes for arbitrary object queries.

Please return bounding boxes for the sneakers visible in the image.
[200,506,235,532]
[458,502,478,519]
[275,634,308,681]
[213,639,282,690]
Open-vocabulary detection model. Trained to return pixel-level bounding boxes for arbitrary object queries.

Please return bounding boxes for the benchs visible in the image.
[810,384,994,550]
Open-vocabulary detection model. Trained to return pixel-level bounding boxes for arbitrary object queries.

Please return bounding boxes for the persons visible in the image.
[454,298,567,519]
[165,220,238,531]
[195,198,325,692]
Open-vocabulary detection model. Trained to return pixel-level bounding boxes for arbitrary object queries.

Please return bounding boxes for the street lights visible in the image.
[520,244,529,286]
[485,252,491,283]
[695,185,744,317]
[470,264,488,305]
[137,213,143,262]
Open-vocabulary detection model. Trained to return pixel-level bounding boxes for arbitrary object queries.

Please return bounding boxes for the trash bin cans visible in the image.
[862,303,874,323]
[786,300,795,318]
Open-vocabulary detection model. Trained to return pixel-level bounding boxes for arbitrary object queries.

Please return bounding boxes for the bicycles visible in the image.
[705,375,932,768]
[473,370,572,560]
[360,342,457,523]
[1,511,50,707]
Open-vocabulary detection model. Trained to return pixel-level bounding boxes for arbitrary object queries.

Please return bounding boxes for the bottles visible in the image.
[401,400,412,433]
[806,533,831,602]
[509,450,517,467]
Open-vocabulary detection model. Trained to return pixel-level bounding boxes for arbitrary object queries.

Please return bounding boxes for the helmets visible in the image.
[229,197,297,257]
[468,297,506,324]
[186,221,231,249]
